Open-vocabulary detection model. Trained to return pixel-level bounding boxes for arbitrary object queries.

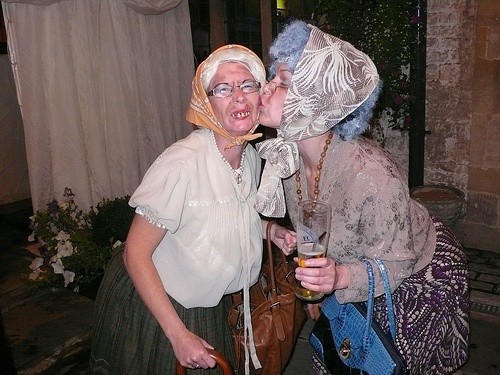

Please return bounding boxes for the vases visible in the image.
[409,184,464,228]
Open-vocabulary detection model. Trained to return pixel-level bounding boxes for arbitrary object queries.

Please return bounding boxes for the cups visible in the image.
[294,200,331,301]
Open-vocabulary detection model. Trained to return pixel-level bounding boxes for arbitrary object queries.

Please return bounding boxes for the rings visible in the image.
[194,364,201,369]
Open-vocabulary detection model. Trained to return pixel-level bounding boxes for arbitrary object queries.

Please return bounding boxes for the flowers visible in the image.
[20,185,122,297]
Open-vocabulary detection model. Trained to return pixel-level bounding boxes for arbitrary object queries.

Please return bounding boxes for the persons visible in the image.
[91,43,299,374]
[256,19,470,375]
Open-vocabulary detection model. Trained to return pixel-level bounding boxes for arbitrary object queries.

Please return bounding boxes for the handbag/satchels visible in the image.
[228,222,306,375]
[309,256,407,375]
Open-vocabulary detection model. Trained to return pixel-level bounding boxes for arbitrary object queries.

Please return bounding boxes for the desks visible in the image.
[0,271,95,375]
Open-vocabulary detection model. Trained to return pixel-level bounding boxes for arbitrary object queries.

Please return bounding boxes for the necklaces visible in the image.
[211,129,248,185]
[293,127,336,229]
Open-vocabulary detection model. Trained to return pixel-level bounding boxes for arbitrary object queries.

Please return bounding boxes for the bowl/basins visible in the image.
[410,184,465,228]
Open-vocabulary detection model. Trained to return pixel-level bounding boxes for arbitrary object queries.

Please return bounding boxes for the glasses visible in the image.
[265,80,289,92]
[206,81,262,98]
